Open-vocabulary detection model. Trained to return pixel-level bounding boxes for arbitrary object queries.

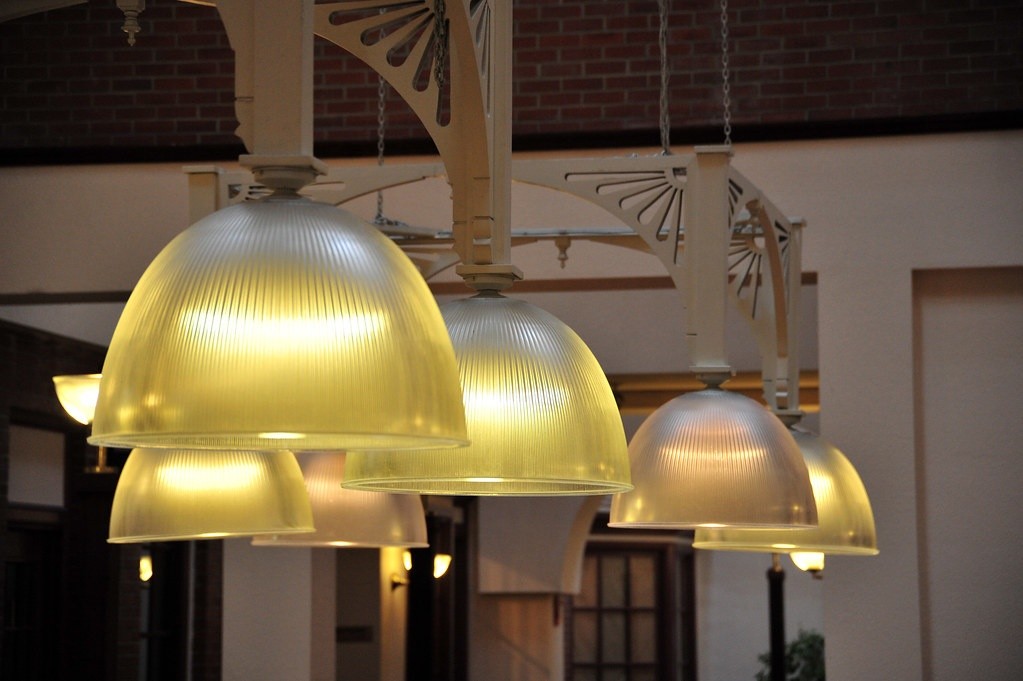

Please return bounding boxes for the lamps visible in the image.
[250,453,431,550]
[788,551,825,574]
[85,182,470,452]
[339,289,633,497]
[53,374,102,425]
[108,446,318,544]
[607,383,819,529]
[691,415,880,557]
[390,552,452,591]
